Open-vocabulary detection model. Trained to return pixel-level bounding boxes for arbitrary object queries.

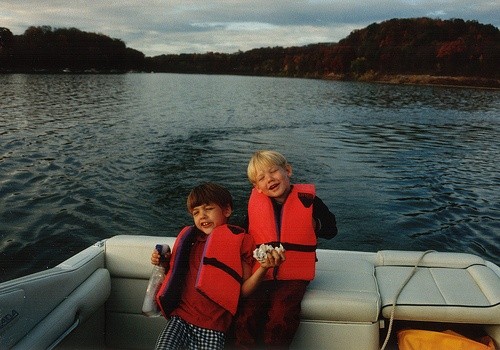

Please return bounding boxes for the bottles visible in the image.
[142,244,171,316]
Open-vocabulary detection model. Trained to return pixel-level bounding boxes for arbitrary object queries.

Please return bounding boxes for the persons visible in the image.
[150,183,287,350]
[225,149,338,350]
